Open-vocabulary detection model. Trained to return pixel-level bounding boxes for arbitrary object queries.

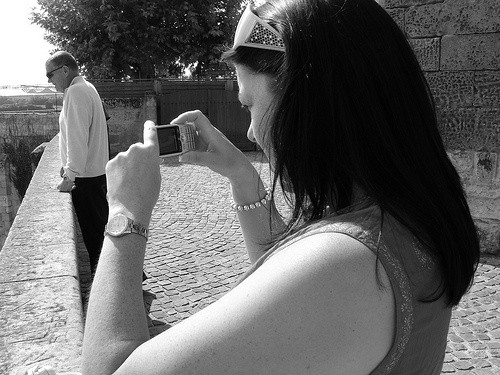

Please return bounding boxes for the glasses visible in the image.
[232,3,287,51]
[46,63,66,79]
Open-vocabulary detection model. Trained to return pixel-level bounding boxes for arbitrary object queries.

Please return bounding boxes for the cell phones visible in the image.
[154,123,198,158]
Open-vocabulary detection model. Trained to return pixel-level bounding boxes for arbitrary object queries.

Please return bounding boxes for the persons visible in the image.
[83,0,480,375]
[45,51,147,288]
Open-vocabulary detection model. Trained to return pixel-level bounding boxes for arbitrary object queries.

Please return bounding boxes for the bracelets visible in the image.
[231,187,272,211]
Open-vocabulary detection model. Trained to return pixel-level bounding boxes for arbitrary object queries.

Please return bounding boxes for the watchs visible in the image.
[104,212,148,241]
[62,174,72,183]
